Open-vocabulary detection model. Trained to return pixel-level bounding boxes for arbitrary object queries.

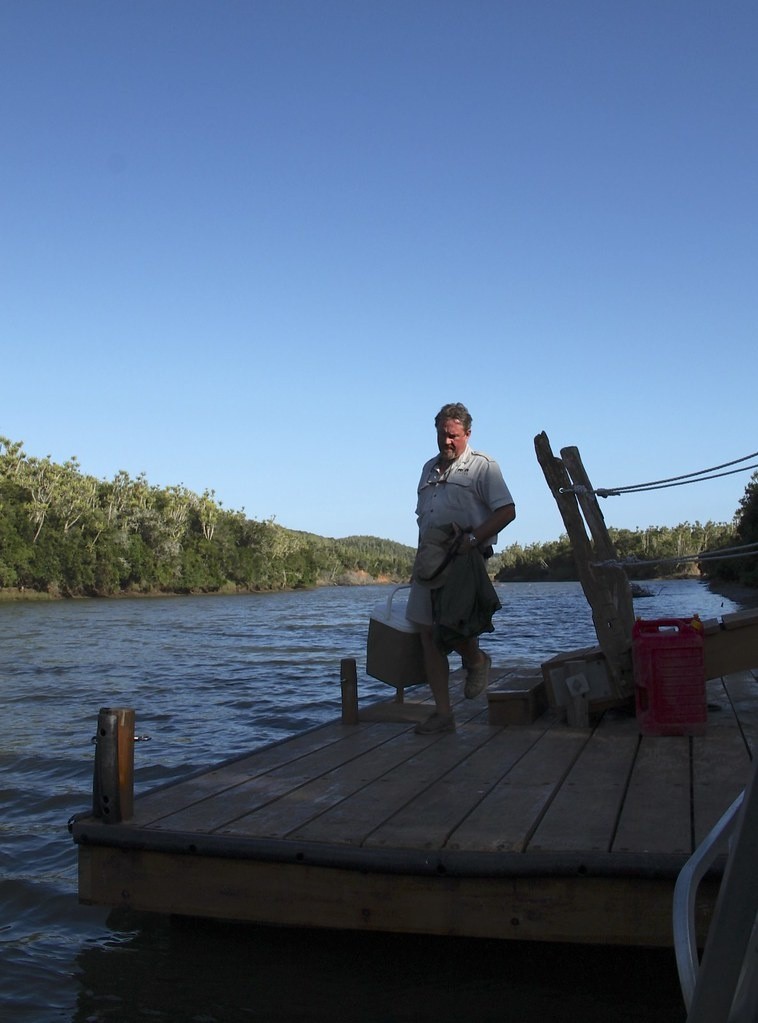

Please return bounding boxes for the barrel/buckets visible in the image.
[632,615,708,737]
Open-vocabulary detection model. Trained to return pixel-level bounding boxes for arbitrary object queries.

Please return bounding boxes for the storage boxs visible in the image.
[367,584,427,688]
[632,613,706,737]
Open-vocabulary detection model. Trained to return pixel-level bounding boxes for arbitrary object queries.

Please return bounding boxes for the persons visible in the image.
[406,402,516,735]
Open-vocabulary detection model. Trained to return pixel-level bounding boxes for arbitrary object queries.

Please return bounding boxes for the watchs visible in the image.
[469,534,477,546]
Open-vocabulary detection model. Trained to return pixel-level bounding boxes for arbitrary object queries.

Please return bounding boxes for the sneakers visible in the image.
[414,710,457,735]
[464,651,492,701]
